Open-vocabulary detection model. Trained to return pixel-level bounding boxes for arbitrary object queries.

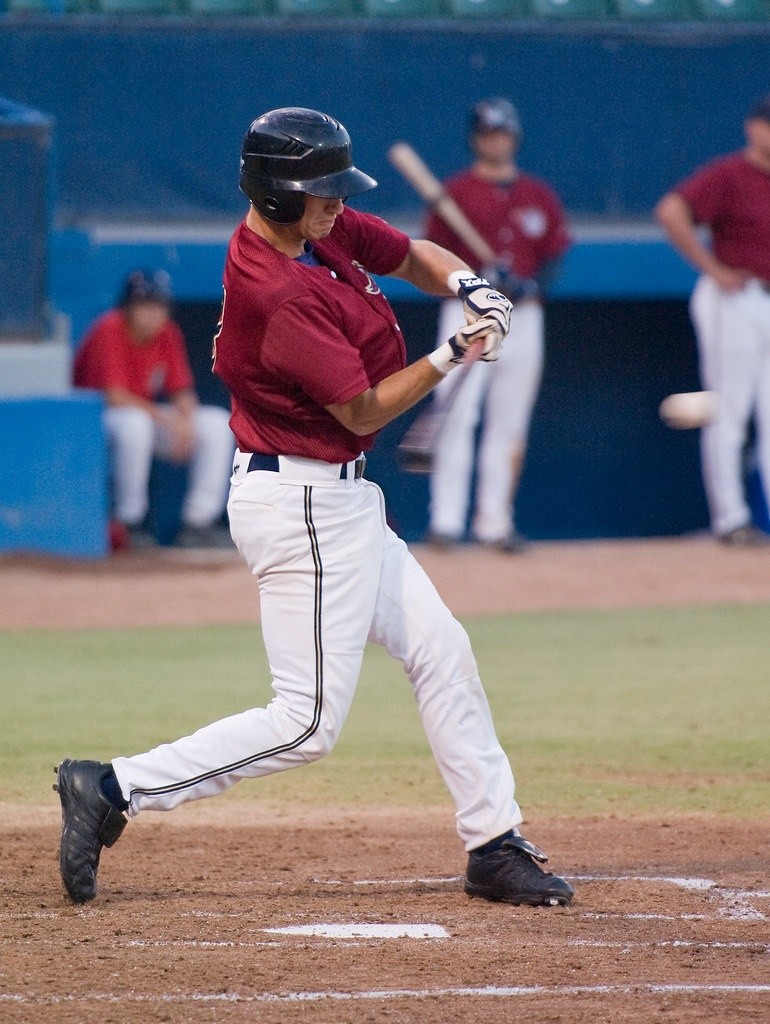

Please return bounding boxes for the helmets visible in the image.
[238,106,378,225]
[118,268,175,304]
[473,98,521,135]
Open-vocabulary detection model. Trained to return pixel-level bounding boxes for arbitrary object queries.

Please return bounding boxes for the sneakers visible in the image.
[52,758,128,905]
[464,836,574,907]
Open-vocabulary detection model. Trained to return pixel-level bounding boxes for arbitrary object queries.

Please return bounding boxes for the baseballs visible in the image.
[659,393,711,429]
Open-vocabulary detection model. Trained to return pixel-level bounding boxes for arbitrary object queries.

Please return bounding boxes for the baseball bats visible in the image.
[385,141,498,263]
[399,334,485,475]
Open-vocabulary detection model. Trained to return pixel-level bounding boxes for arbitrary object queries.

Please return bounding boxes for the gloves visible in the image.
[449,273,512,338]
[427,319,502,374]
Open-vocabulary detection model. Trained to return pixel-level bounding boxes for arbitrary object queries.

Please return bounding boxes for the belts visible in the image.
[248,452,367,481]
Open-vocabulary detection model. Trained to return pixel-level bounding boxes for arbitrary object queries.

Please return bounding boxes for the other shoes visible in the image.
[717,525,769,550]
[168,525,236,548]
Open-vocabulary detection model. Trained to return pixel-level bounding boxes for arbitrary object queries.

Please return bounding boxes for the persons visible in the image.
[424,96,571,558]
[70,269,235,552]
[653,103,770,550]
[54,109,575,906]
